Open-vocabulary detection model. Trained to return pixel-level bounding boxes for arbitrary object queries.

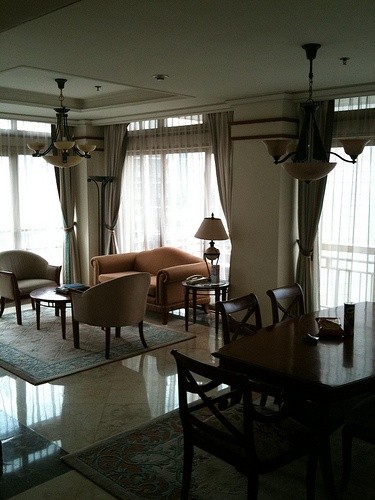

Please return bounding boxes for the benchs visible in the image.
[90,246,212,325]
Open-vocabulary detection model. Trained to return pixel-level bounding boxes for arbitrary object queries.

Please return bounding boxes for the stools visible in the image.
[340,395,375,500]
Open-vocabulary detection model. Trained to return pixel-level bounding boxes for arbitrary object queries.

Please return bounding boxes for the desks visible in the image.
[210,301,375,500]
[30,285,71,340]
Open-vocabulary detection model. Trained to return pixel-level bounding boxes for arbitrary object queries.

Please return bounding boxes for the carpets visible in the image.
[60,387,375,500]
[0,306,197,385]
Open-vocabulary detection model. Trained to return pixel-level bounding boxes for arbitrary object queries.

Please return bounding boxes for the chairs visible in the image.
[67,272,151,360]
[169,349,334,500]
[266,282,305,324]
[0,250,62,326]
[216,292,262,345]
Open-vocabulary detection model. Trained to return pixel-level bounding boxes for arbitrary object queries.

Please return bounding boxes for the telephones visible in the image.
[186,273,212,285]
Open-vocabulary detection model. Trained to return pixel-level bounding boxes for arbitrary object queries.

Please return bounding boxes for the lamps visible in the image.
[261,43,371,185]
[27,79,96,168]
[193,213,229,282]
[87,176,113,256]
